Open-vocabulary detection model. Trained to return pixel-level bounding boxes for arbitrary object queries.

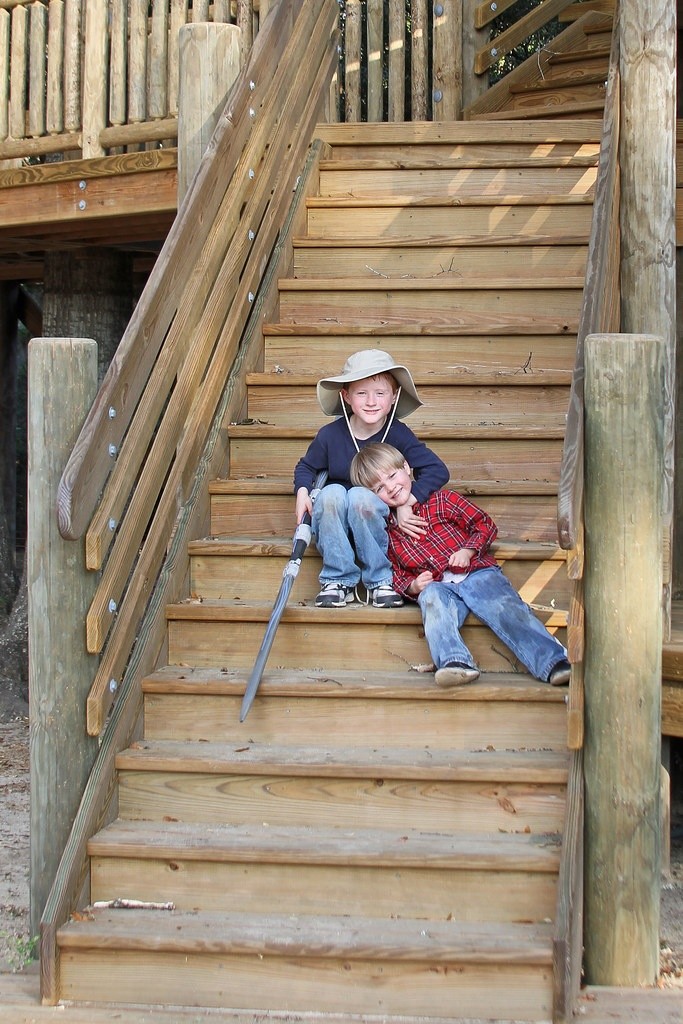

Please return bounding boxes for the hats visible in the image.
[316,349,424,420]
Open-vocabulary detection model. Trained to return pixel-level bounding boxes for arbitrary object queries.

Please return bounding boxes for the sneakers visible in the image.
[363,585,404,608]
[314,581,365,607]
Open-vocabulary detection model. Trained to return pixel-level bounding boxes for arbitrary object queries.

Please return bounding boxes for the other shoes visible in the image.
[549,661,571,685]
[435,661,478,686]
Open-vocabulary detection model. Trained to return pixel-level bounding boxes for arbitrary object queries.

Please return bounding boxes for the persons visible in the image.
[293,349,450,609]
[349,442,573,686]
